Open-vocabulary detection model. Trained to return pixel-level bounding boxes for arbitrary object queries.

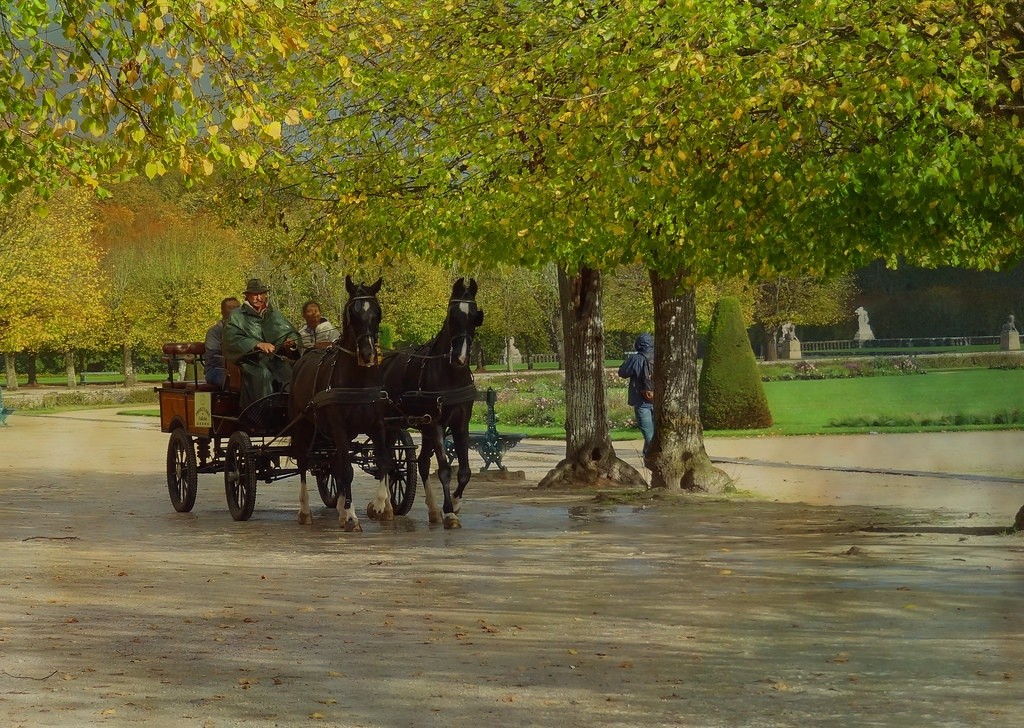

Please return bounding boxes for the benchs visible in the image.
[159,341,221,437]
[446,386,526,472]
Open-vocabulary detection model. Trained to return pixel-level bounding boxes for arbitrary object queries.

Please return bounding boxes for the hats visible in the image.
[242,278,268,294]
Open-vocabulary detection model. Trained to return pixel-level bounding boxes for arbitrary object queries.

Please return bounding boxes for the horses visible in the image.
[366,276,484,530]
[277,274,394,533]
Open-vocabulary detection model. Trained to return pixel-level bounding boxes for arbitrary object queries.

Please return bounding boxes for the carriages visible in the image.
[152,274,485,530]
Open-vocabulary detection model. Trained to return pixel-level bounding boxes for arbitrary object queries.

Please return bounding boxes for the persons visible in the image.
[204,279,341,424]
[618,334,654,454]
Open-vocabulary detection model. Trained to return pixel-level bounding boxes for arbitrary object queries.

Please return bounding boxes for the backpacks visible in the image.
[629,353,654,404]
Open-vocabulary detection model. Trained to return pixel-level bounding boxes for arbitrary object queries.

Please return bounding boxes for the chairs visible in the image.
[222,358,240,393]
